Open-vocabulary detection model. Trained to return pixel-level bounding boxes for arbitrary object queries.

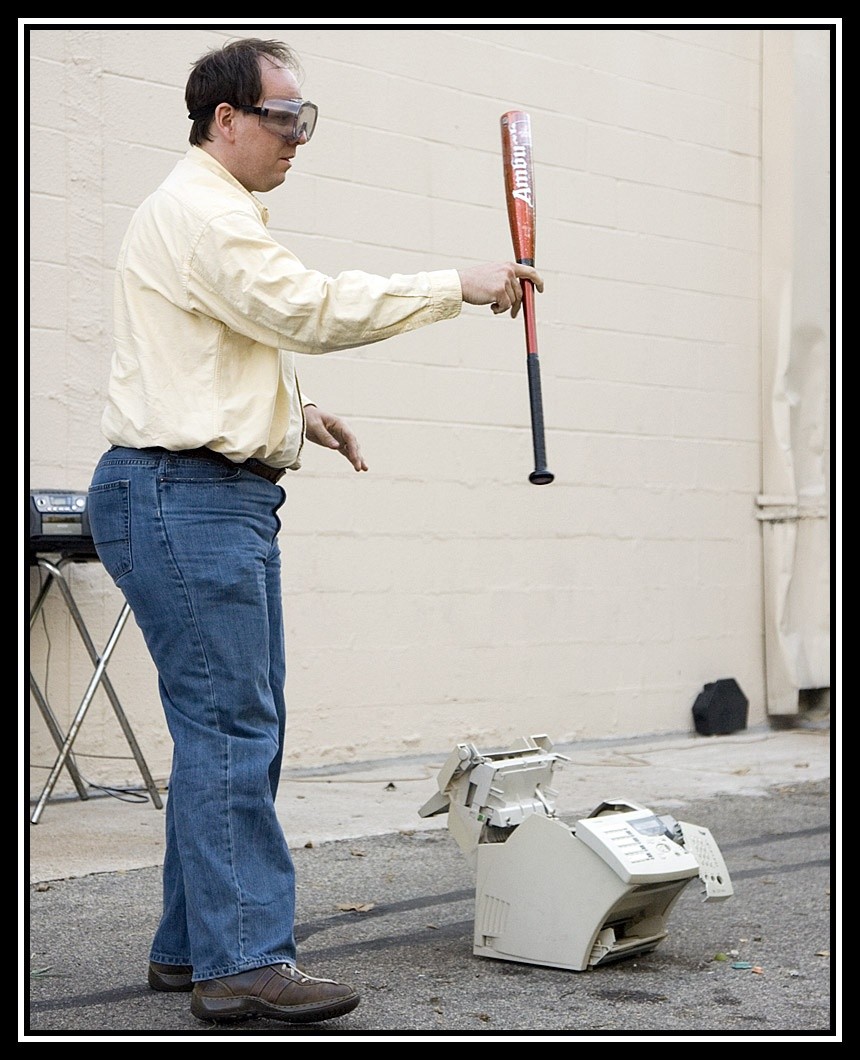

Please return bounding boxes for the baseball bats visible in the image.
[499,111,554,485]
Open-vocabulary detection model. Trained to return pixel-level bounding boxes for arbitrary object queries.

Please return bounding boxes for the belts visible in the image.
[192,447,285,485]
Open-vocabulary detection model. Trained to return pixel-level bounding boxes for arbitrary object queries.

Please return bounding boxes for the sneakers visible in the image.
[149,955,197,992]
[190,957,360,1024]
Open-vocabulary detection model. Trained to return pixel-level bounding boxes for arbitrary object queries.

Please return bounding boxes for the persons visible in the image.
[87,38,544,1023]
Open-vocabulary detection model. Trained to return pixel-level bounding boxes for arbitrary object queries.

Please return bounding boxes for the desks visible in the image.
[22,533,164,825]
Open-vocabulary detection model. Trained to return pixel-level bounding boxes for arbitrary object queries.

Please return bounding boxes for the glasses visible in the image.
[258,96,318,144]
